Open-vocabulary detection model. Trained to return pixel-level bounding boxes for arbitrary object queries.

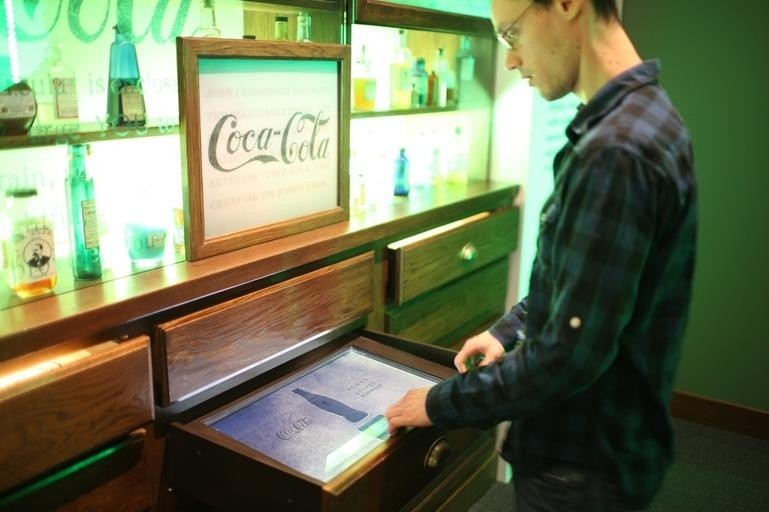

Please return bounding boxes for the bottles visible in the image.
[393,148,411,197]
[292,387,369,425]
[103,32,146,127]
[46,42,80,128]
[273,9,311,43]
[352,28,460,113]
[1,137,186,300]
[191,5,222,38]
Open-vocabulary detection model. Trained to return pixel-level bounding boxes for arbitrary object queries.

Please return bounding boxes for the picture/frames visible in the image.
[183,334,462,486]
[173,36,353,262]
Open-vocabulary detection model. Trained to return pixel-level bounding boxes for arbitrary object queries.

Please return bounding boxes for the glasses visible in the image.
[499,3,541,50]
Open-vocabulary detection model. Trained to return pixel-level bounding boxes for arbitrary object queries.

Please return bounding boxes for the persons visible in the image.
[383,0,699,512]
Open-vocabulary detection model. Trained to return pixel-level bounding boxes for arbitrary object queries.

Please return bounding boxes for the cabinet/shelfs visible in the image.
[0,0,531,511]
